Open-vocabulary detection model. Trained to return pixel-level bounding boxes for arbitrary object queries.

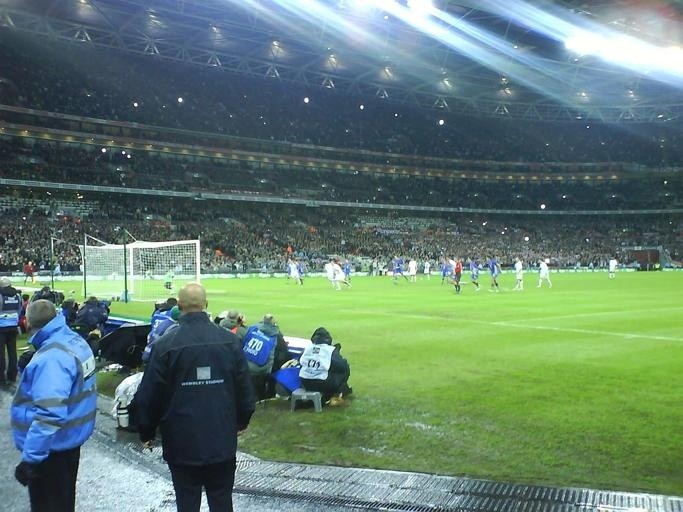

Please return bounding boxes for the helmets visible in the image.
[156,300,166,309]
[170,305,181,320]
[0,276,11,288]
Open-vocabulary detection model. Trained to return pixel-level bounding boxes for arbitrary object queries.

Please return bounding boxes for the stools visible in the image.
[290,387,324,413]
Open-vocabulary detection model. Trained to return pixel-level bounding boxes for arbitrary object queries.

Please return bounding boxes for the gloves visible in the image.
[15,461,36,487]
